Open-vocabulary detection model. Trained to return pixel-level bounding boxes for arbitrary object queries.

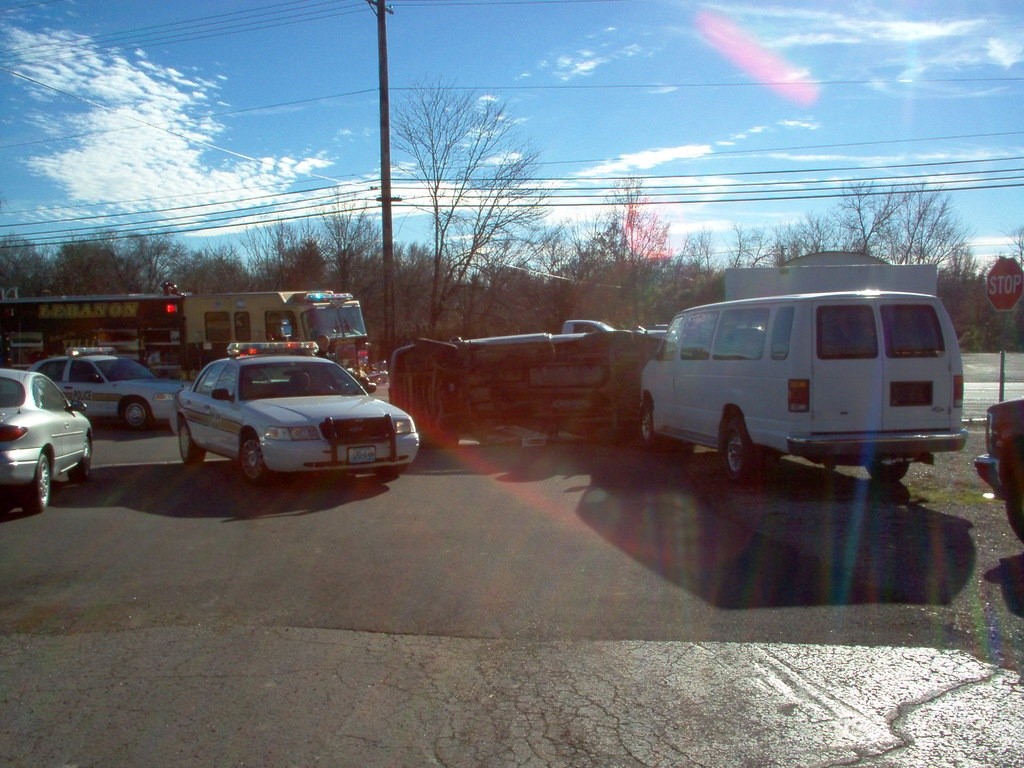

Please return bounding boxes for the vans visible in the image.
[637,288,969,487]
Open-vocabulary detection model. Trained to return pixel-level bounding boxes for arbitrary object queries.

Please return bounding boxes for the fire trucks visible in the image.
[0,276,374,380]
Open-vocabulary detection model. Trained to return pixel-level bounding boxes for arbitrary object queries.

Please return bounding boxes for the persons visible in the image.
[315,334,330,359]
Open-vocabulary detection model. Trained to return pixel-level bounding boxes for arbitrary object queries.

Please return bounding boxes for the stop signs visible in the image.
[985,258,1024,312]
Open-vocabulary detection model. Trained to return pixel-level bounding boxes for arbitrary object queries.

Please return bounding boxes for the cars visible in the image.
[0,365,95,516]
[972,395,1024,547]
[26,345,196,431]
[386,318,672,451]
[172,337,421,489]
